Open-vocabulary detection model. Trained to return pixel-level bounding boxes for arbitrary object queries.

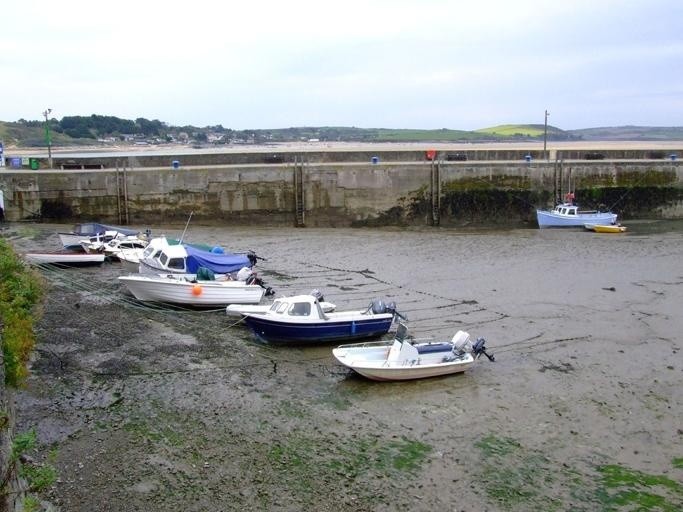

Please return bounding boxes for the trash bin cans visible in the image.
[31,159,38,170]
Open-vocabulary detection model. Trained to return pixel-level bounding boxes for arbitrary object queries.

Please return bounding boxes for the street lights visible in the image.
[42,109,53,168]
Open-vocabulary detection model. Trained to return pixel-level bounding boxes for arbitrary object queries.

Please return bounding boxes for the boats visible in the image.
[118,236,265,306]
[57,221,150,264]
[584,221,628,233]
[332,330,486,381]
[536,204,618,227]
[16,253,105,265]
[225,295,396,345]
